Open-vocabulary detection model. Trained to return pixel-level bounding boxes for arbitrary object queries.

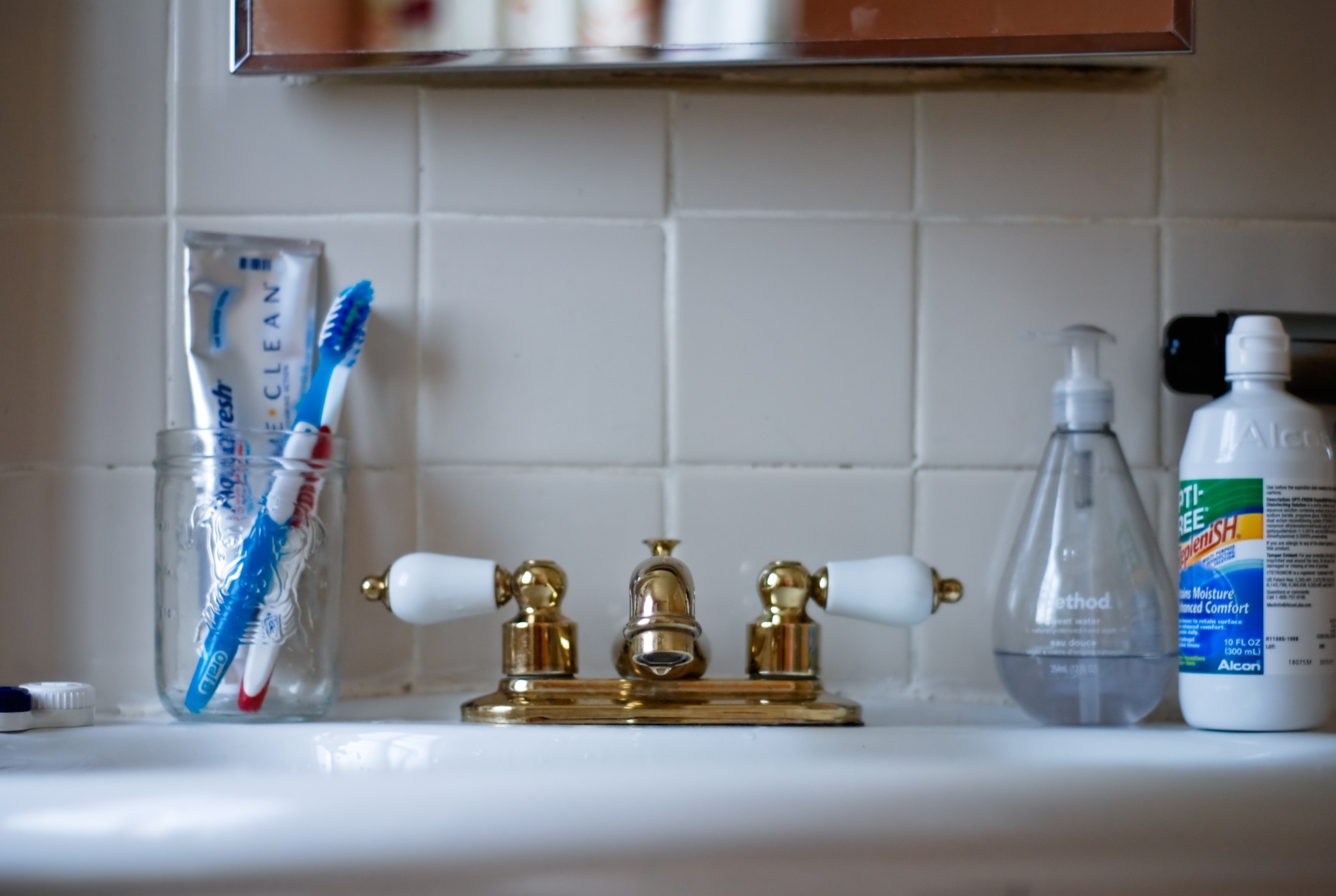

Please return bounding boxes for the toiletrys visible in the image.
[985,311,1335,731]
[176,223,328,683]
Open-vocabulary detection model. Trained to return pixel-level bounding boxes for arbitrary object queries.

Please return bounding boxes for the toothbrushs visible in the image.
[177,277,376,716]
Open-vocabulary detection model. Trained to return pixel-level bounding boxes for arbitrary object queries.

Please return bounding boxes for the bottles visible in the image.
[1179,314,1335,733]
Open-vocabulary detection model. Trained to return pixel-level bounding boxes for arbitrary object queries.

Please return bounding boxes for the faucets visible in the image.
[608,538,713,683]
